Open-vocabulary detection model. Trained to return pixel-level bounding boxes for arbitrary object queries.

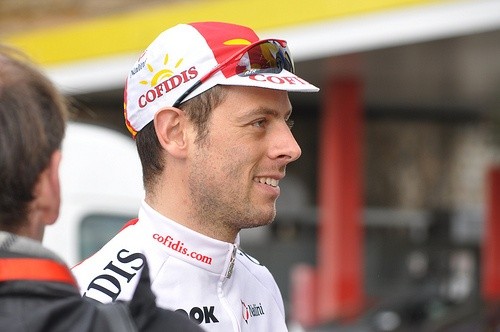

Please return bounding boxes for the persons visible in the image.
[69,22,320,332]
[0,50,208,332]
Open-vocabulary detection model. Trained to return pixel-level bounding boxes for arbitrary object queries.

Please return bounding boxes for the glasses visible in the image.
[173,38,295,107]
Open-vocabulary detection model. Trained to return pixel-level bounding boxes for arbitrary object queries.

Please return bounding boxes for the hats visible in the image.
[123,22,319,138]
